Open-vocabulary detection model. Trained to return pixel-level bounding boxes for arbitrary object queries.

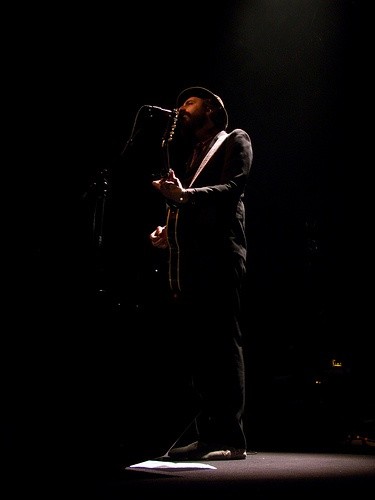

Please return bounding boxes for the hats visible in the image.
[178,88,229,130]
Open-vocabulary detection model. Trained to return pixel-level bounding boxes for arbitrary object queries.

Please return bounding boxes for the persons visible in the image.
[148,86,252,459]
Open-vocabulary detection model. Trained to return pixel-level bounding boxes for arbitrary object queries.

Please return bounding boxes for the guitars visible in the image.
[160,107,191,293]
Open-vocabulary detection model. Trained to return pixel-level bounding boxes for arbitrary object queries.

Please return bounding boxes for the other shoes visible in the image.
[164,440,248,460]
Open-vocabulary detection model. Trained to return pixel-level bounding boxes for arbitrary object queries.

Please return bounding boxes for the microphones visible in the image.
[143,104,182,120]
